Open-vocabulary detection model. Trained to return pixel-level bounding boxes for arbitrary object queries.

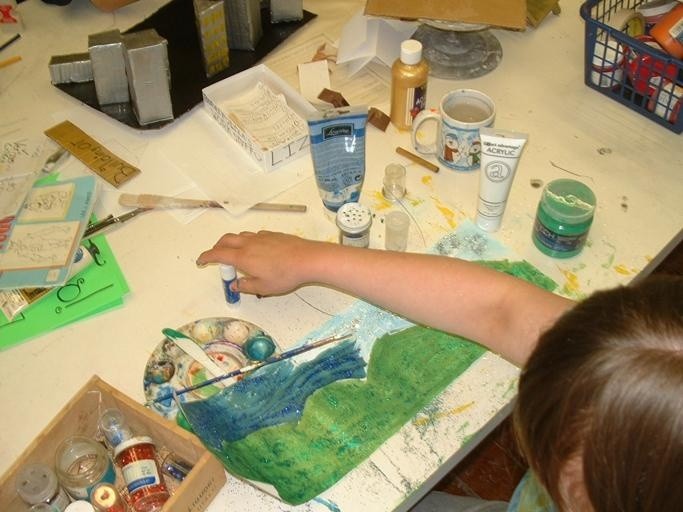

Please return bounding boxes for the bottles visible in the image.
[220,262,241,306]
[589,0,683,123]
[334,202,371,249]
[390,38,428,131]
[531,177,598,259]
[385,211,410,253]
[383,162,406,201]
[13,407,171,512]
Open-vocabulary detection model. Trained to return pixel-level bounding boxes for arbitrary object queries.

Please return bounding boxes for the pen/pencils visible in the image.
[1,33,24,68]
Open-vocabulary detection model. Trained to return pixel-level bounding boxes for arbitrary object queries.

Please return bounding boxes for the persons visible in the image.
[195,229,683,512]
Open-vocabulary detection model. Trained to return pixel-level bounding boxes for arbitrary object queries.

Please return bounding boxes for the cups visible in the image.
[412,88,496,170]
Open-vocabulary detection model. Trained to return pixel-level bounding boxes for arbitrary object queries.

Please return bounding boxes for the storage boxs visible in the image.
[363,1,561,33]
[2,375,227,511]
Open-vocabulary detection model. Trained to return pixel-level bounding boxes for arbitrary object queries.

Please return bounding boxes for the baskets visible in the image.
[580,0,682,136]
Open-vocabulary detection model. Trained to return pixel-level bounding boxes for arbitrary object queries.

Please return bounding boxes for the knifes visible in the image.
[162,327,235,388]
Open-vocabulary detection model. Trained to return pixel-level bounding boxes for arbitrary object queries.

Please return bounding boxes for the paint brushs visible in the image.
[83,208,153,237]
[119,194,307,212]
[145,329,353,406]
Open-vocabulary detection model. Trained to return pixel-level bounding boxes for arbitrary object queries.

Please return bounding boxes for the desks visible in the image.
[1,1,683,512]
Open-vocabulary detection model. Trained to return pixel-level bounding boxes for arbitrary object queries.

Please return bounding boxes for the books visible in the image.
[0,175,99,288]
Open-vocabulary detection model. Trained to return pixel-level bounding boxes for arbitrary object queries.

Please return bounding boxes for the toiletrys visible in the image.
[476,127,529,234]
[307,105,372,212]
